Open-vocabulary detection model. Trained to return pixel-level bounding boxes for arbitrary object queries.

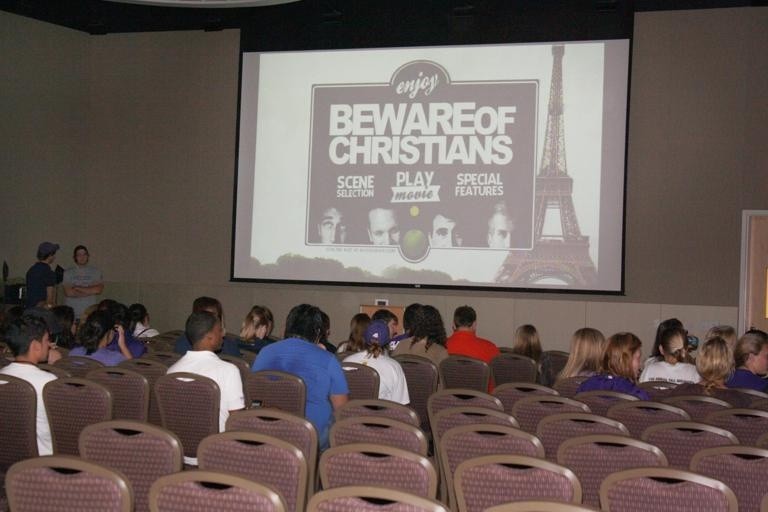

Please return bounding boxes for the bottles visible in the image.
[688,335,704,347]
[75,313,80,333]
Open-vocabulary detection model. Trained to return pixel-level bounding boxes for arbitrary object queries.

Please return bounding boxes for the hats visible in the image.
[22,307,64,334]
[37,242,59,256]
[365,319,389,346]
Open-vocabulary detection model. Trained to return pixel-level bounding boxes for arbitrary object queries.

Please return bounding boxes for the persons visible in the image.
[427,206,480,248]
[512,325,554,391]
[484,199,529,248]
[638,319,767,409]
[2,293,160,456]
[165,296,505,465]
[316,200,360,245]
[554,328,654,401]
[366,202,403,246]
[25,242,60,308]
[61,244,105,324]
